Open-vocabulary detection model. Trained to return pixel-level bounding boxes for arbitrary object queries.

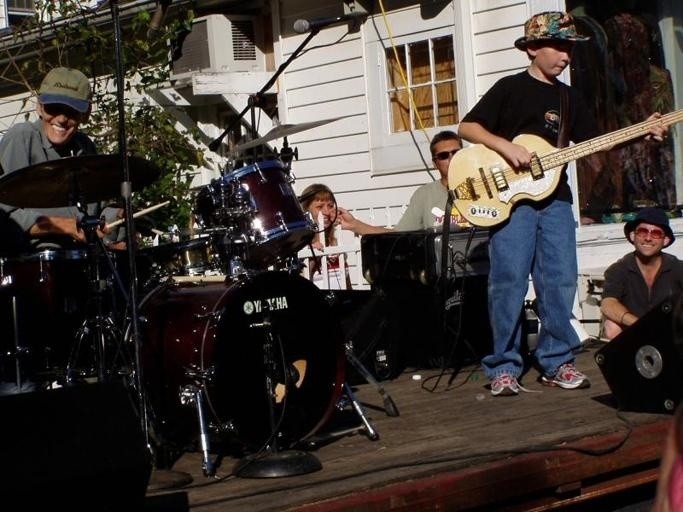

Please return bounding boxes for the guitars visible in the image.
[447,107,683,229]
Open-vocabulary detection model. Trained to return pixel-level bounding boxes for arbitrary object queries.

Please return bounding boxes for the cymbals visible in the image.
[230,113,366,151]
[0,155,160,208]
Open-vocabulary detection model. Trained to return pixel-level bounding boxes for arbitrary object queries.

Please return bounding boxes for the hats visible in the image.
[623,206,675,250]
[514,11,592,52]
[39,67,92,113]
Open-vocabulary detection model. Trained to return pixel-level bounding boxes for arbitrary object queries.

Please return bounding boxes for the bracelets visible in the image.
[619,310,633,325]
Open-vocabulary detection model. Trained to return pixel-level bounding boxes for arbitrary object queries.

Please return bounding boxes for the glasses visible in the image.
[433,149,460,161]
[634,227,667,240]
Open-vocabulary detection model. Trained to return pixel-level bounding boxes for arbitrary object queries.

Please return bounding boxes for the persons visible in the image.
[598,204,683,341]
[290,182,341,252]
[325,129,473,236]
[0,65,143,253]
[457,12,670,398]
[649,377,681,510]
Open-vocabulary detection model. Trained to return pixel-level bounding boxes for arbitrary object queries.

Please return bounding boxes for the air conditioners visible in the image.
[164,14,266,82]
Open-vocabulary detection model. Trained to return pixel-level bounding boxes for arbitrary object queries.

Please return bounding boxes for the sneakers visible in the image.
[144,465,192,490]
[490,374,519,396]
[541,363,592,390]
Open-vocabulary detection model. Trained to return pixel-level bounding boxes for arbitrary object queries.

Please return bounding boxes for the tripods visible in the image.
[59,200,130,391]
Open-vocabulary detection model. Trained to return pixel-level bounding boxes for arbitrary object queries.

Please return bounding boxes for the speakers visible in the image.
[591,291,683,413]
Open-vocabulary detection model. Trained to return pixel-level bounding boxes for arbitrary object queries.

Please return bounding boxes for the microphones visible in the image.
[291,10,367,35]
[262,352,299,384]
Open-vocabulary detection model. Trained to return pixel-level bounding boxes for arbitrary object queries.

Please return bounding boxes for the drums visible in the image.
[0,250,133,397]
[198,159,316,270]
[136,238,208,281]
[136,273,347,456]
